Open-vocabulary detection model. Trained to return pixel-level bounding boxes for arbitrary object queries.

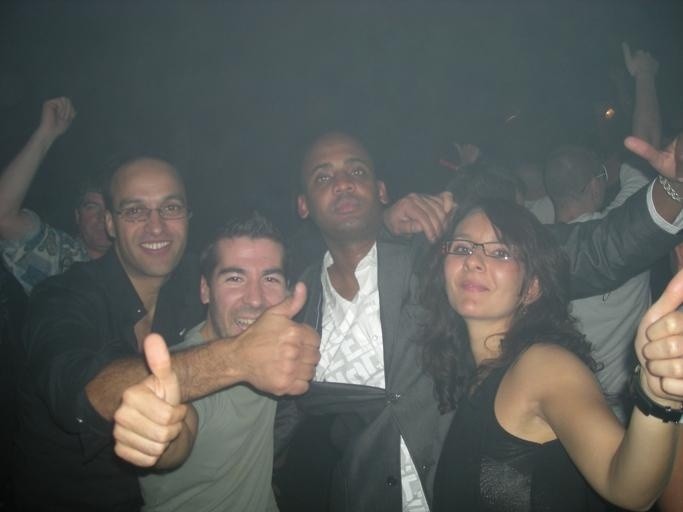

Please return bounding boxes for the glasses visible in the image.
[114,205,190,222]
[441,237,517,261]
[581,164,608,192]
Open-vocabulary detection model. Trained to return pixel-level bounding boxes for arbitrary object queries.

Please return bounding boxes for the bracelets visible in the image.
[658,173,680,200]
[629,362,683,423]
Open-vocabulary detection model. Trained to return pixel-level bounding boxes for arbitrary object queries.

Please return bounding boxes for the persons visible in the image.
[417,195,683,512]
[20,154,318,512]
[112,210,292,511]
[528,43,662,512]
[272,131,683,511]
[0,98,556,300]
